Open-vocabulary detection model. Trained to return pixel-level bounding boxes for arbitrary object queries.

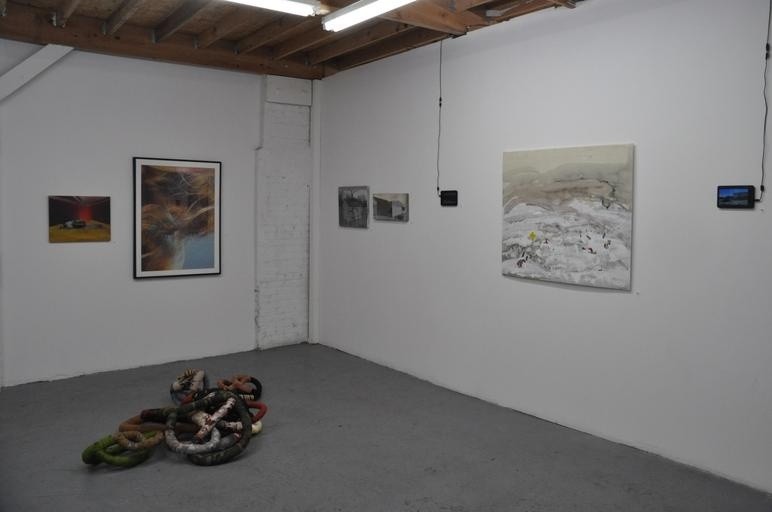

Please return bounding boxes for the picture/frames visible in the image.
[131,152,223,282]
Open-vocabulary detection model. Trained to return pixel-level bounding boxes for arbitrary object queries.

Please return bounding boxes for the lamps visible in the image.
[230,0,415,34]
